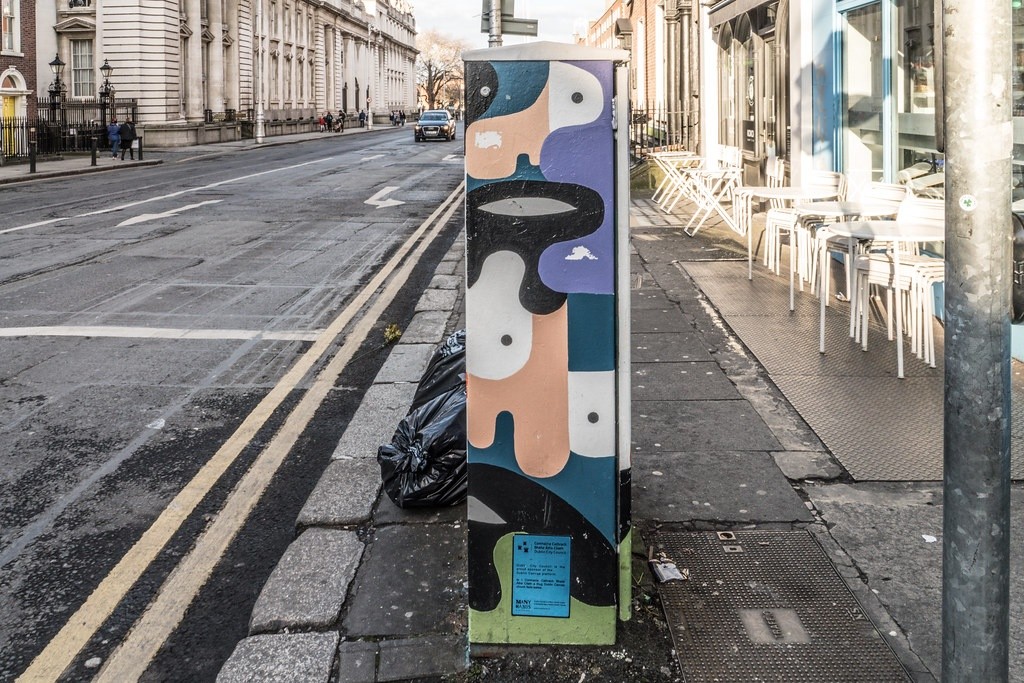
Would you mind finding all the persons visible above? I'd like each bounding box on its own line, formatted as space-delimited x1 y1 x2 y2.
390 110 406 126
117 118 136 160
359 110 366 127
338 110 346 132
320 113 325 132
325 112 333 132
107 118 121 159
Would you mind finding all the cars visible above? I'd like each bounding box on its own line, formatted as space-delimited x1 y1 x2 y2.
414 109 457 142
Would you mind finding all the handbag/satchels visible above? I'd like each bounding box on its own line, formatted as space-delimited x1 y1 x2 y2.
131 139 139 149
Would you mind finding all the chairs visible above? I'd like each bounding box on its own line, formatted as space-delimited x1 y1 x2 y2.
646 141 945 377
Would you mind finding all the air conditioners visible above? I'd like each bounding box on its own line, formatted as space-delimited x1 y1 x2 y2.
622 36 632 48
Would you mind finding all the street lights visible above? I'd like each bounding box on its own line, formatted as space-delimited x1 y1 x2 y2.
49 53 66 124
99 59 115 127
368 23 385 125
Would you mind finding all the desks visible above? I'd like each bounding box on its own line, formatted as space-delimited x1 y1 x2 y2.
789 201 895 312
748 188 836 282
648 150 696 203
819 220 944 378
660 156 712 213
679 169 746 237
